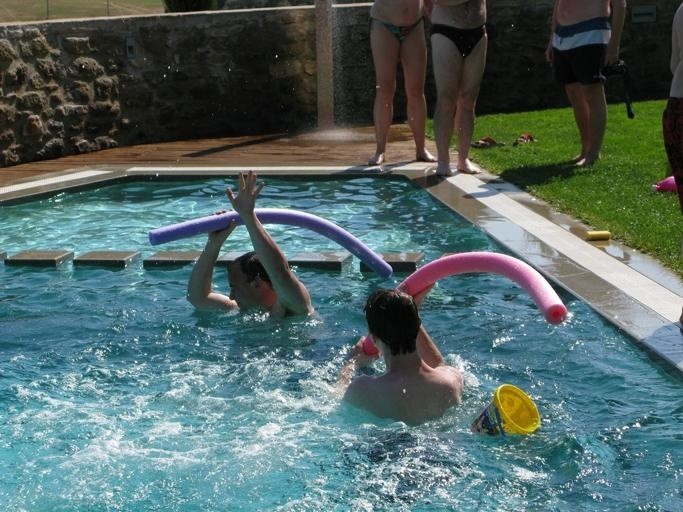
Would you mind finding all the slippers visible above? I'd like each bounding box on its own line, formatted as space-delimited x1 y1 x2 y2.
515 133 539 146
471 135 506 149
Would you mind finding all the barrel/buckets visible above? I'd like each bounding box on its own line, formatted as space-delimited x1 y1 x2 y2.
474 384 541 436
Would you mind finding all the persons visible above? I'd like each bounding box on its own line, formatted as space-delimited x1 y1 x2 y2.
429 1 489 177
367 0 438 167
544 0 627 171
661 4 683 214
185 170 312 323
335 289 463 428
